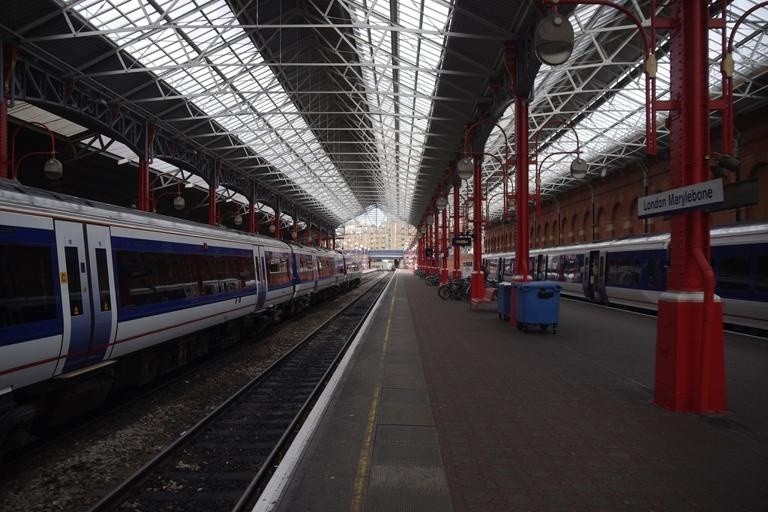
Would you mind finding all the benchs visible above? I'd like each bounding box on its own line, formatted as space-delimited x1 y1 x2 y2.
470 287 496 311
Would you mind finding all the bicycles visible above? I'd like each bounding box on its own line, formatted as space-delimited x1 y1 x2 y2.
437 276 470 300
414 270 440 286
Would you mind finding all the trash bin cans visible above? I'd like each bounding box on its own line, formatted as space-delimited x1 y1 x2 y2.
514 281 562 334
497 282 511 322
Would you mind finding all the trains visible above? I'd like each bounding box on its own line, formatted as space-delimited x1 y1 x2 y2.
0 178 361 397
470 222 767 336
